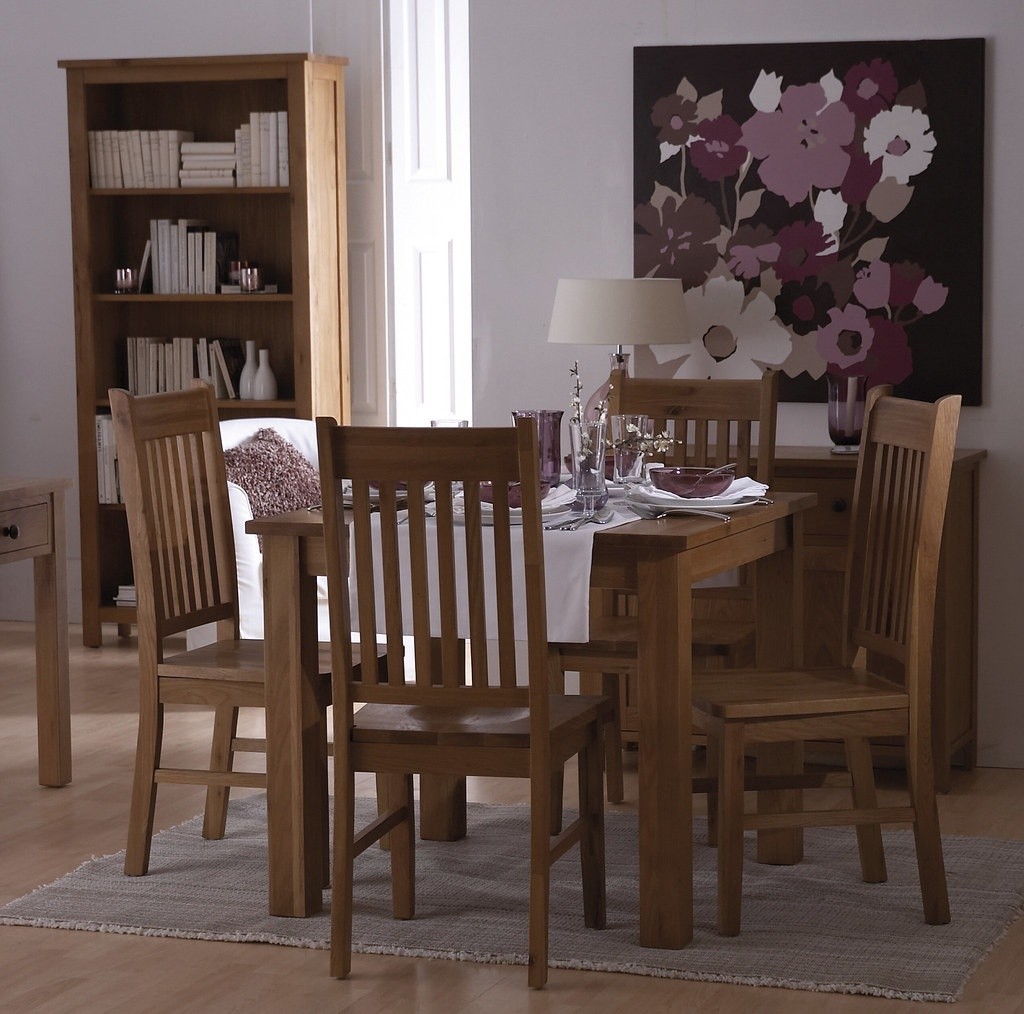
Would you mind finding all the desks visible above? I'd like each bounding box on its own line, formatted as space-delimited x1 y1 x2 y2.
0 479 74 788
244 475 820 950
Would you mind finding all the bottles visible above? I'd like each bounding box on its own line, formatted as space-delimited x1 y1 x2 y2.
238 340 258 400
254 349 278 400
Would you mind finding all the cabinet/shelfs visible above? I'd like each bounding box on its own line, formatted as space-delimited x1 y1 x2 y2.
564 446 988 793
57 54 352 649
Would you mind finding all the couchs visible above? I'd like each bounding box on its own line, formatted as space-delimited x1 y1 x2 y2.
188 418 416 682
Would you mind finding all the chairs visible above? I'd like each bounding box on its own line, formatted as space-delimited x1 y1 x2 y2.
315 417 608 991
690 383 963 937
106 383 406 875
542 368 778 846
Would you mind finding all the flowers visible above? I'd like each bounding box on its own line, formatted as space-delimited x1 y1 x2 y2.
570 360 682 475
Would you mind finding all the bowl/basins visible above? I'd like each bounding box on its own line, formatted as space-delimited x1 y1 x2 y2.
564 455 614 480
649 466 735 498
479 481 550 506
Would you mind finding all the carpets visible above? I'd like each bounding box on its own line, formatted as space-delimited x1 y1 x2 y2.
0 789 1024 1001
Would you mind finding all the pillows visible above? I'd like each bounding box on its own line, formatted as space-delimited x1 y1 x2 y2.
222 429 324 554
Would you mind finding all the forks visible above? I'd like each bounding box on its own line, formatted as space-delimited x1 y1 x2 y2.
627 504 731 523
569 497 594 531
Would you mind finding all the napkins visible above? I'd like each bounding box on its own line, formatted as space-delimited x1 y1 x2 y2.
627 476 768 500
455 483 577 512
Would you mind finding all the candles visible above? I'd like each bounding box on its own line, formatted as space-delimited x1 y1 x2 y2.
845 377 857 437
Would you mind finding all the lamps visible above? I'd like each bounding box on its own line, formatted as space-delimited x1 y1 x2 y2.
547 277 689 448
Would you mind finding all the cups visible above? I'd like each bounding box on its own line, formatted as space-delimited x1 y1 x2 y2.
512 409 565 486
611 414 648 482
114 267 137 294
570 422 608 498
225 260 249 285
824 374 869 446
239 268 261 293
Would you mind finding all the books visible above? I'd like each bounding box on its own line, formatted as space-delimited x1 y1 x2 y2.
126 337 239 399
88 111 289 189
113 585 137 606
136 218 237 294
95 415 126 504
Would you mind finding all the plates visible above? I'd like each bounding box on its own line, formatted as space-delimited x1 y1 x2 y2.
640 489 744 505
608 487 626 498
343 490 430 503
453 484 577 513
624 494 759 514
453 507 573 525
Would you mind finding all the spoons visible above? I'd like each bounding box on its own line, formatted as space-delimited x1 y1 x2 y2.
558 507 614 532
